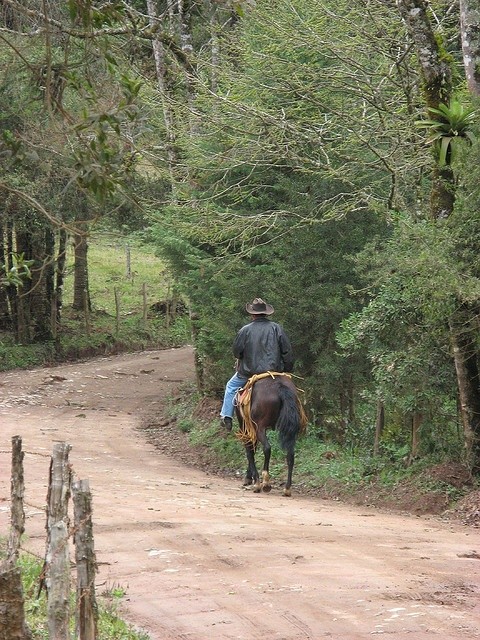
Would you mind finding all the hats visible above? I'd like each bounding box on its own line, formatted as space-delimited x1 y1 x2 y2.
246 298 274 315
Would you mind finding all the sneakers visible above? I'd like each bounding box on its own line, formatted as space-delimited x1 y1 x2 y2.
220 417 232 430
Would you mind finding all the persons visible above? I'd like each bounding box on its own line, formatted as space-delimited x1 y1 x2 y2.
219 297 295 431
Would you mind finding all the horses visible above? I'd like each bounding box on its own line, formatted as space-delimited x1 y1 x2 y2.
233 358 301 496
